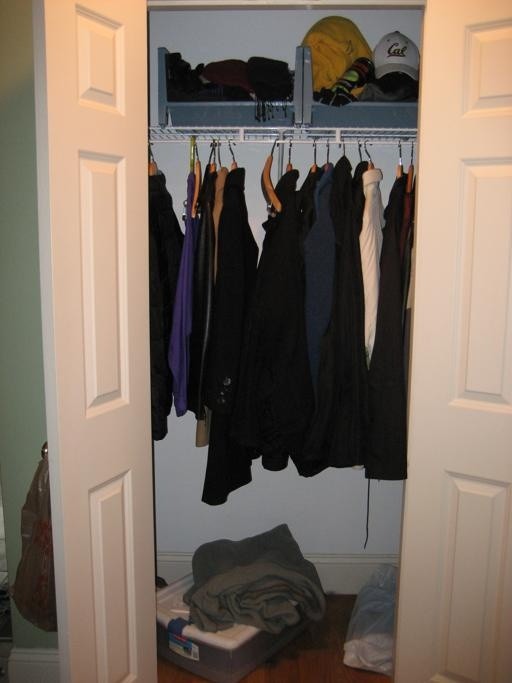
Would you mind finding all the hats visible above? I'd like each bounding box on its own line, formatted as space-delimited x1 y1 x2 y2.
374 30 426 83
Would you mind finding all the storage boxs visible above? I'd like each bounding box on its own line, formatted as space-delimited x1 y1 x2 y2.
155 572 307 683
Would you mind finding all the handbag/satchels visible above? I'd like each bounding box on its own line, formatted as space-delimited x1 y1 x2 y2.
13 459 59 633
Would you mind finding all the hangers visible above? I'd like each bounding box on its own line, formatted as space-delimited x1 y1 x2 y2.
150 126 416 220
